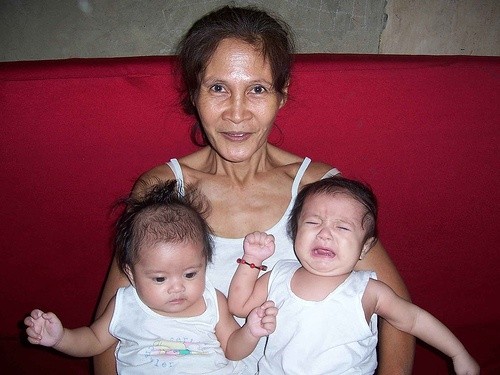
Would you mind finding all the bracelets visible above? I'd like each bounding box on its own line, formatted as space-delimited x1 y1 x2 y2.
235 257 269 273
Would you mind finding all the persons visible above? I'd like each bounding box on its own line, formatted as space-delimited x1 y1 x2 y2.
24 179 278 375
90 4 415 375
226 176 481 375
